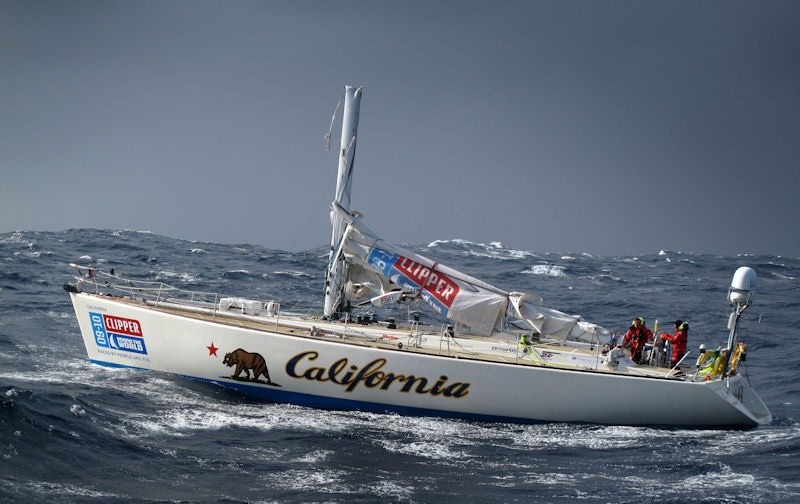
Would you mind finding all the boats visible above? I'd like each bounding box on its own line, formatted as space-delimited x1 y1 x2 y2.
63 85 773 430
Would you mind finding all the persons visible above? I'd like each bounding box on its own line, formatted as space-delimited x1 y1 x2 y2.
660 319 689 369
622 317 653 364
602 343 611 356
343 300 352 323
387 319 396 329
